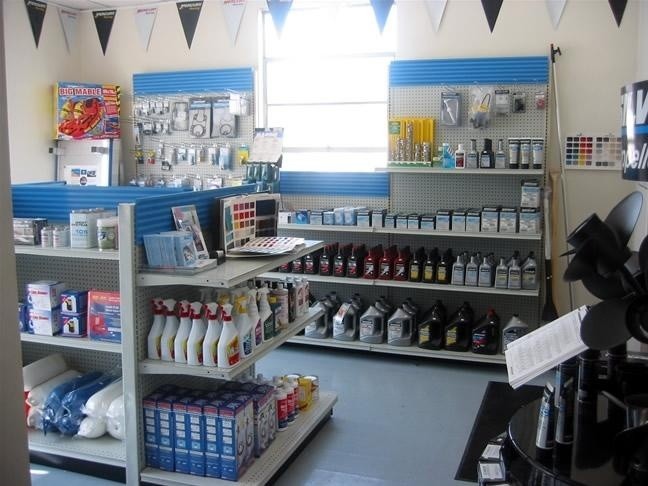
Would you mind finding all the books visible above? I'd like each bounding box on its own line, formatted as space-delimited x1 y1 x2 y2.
142 231 218 275
504 305 591 390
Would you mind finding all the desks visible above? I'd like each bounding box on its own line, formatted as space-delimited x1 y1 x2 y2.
452 378 647 485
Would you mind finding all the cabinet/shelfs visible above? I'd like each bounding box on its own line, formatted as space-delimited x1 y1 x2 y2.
128 183 337 486
9 184 137 484
258 161 548 372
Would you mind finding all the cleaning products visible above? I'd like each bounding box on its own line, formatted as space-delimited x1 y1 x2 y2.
144 272 310 370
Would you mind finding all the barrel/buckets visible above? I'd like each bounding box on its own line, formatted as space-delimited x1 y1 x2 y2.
303 286 529 355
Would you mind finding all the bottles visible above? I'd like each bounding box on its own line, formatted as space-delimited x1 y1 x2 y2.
40 224 69 249
246 276 311 332
274 241 538 292
433 138 506 170
251 372 299 432
394 123 430 163
555 342 628 446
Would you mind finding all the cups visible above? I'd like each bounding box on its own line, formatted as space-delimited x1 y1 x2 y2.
625 392 648 428
